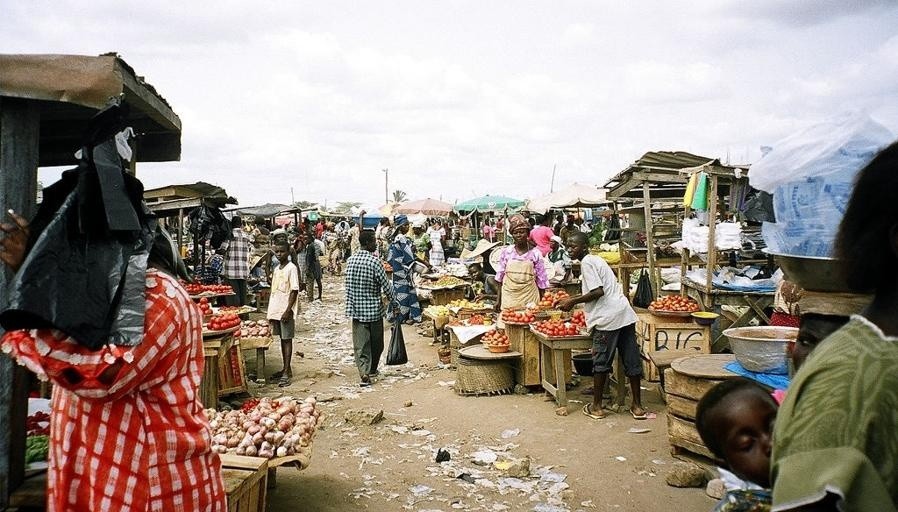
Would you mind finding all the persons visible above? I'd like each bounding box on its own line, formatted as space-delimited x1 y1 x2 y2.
772 313 848 407
0 164 228 511
769 137 898 511
695 378 779 512
559 231 646 421
149 210 360 302
265 240 300 389
346 230 402 386
374 210 623 322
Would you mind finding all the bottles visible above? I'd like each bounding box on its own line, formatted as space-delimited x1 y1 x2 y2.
481 298 494 326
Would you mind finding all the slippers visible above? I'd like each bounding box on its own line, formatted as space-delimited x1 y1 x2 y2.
581 402 606 421
629 405 648 420
359 369 379 387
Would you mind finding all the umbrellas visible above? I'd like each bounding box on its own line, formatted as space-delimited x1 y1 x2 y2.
454 194 525 246
398 198 455 217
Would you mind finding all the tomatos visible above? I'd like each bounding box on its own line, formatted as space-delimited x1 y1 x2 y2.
185 283 233 295
196 296 213 315
207 314 241 337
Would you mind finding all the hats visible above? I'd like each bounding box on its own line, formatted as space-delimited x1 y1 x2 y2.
393 214 409 228
508 214 531 236
464 237 503 260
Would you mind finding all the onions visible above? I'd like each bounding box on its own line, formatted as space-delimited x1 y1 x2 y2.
202 396 323 459
240 320 272 339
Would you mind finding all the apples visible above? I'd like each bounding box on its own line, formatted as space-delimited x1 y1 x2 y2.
502 290 586 336
481 329 510 346
651 294 700 312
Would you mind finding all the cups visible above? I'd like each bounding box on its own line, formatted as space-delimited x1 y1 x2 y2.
532 310 562 322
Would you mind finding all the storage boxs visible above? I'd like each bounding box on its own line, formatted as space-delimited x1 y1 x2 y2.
503 322 571 387
636 312 712 382
219 453 269 512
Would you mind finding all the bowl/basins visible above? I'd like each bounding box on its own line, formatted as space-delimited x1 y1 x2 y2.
571 352 594 376
759 245 846 293
487 344 510 353
691 311 720 325
648 307 698 319
722 325 800 375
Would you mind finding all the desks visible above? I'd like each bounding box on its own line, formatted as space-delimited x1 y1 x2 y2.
422 306 449 345
239 329 272 383
531 330 625 407
203 337 248 409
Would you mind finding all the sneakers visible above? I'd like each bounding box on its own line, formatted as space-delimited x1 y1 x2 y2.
271 369 293 387
